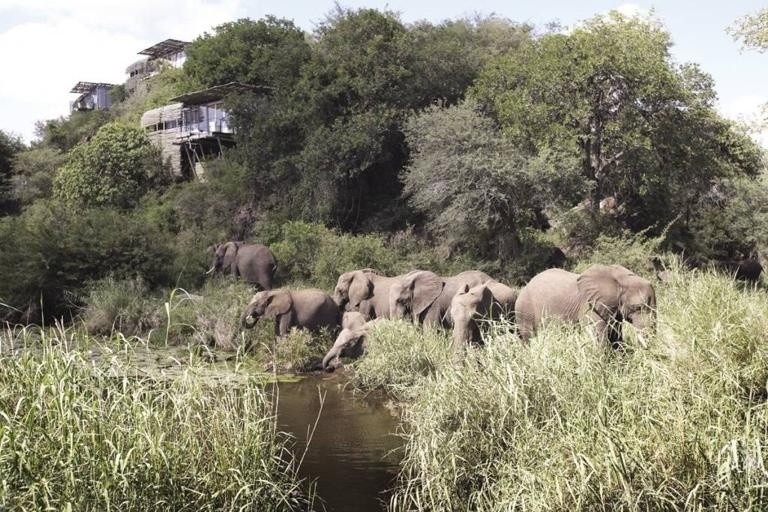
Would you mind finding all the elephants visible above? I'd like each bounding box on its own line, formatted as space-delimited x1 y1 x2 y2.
322 265 656 373
204 242 277 290
241 288 340 338
